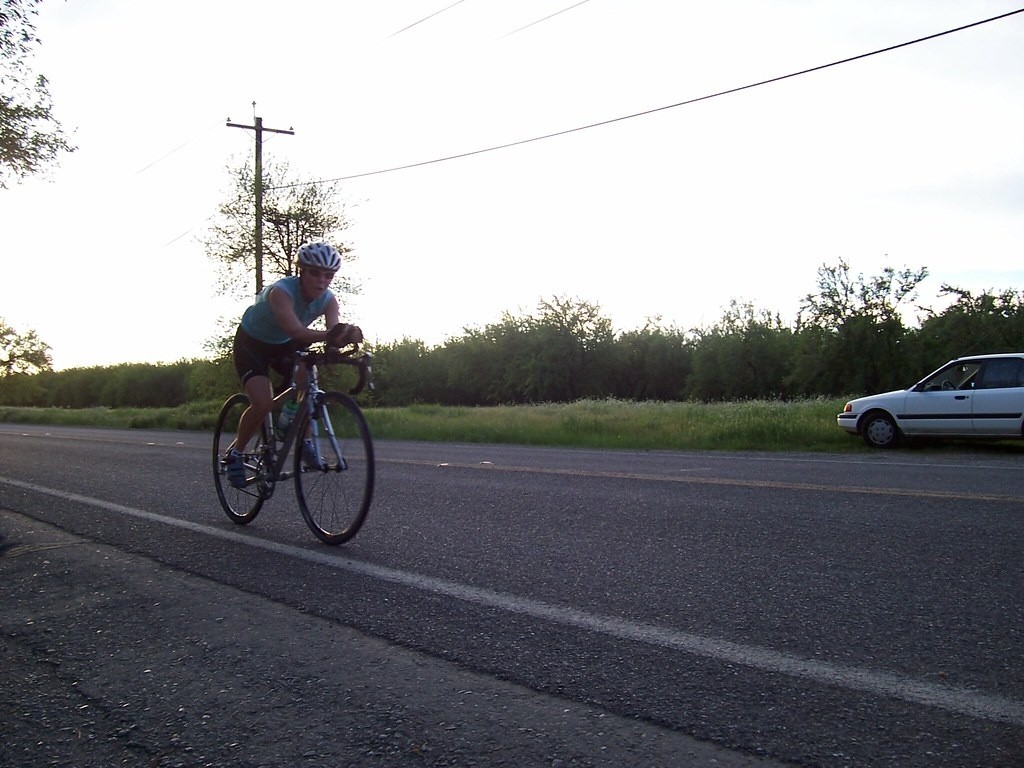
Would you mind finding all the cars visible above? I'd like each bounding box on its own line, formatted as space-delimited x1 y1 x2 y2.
838 353 1024 448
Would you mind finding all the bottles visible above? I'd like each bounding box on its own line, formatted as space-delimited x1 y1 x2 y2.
273 398 299 442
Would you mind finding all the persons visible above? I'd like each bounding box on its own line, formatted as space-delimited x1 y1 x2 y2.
225 244 363 487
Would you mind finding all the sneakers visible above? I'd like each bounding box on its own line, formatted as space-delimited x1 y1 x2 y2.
225 448 246 488
301 439 328 469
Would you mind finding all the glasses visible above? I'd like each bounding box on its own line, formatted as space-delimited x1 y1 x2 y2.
308 270 334 279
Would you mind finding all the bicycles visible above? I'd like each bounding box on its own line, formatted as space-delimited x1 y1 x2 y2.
211 344 377 545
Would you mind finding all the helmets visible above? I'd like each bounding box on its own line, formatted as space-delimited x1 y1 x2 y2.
292 242 341 272
